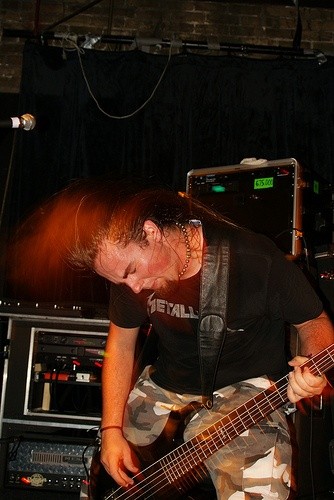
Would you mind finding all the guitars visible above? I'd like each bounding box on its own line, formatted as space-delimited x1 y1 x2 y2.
87 344 334 500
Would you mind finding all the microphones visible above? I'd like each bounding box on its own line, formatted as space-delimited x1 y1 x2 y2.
0 114 36 131
